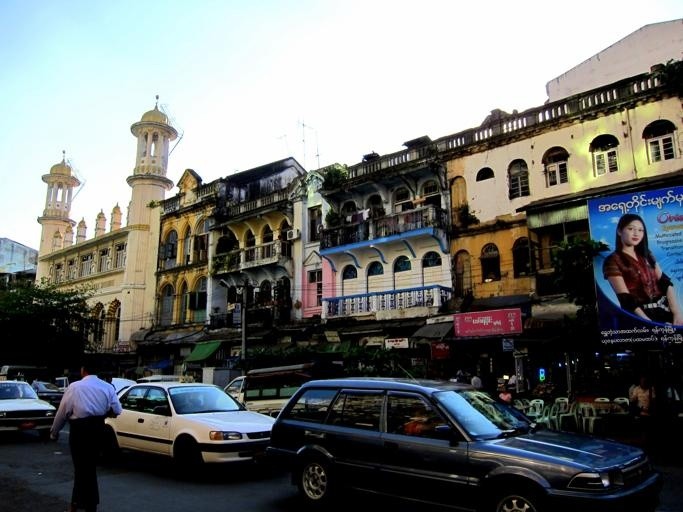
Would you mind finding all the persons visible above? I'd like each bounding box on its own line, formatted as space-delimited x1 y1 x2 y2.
449 363 683 465
401 411 445 437
47 359 123 512
601 212 683 326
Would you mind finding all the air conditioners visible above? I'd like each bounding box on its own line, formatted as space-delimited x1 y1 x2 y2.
287 228 301 240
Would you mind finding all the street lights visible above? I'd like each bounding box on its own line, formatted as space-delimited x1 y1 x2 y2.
219 279 248 376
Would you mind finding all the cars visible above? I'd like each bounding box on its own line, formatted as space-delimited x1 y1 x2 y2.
100 381 276 480
0 365 70 442
224 373 327 419
262 376 659 512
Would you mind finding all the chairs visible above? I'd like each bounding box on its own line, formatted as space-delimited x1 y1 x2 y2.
513 397 631 432
